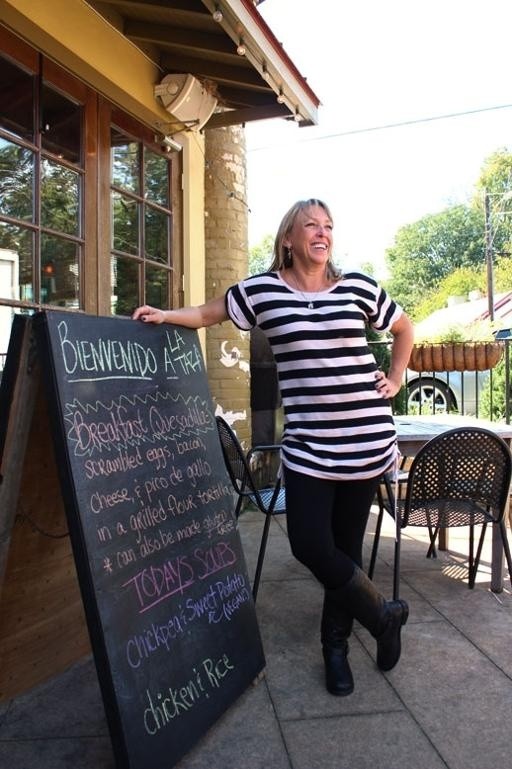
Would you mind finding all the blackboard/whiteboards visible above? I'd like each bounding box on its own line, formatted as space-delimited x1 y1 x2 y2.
46 309 267 767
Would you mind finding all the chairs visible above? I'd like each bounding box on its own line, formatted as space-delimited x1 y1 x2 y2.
217 415 286 606
369 427 512 601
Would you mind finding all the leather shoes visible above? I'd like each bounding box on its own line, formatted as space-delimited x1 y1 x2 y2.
321 632 355 696
377 600 408 671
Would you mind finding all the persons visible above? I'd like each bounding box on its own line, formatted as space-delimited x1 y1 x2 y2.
131 199 414 697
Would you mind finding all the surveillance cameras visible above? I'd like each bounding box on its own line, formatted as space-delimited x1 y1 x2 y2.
156 134 182 155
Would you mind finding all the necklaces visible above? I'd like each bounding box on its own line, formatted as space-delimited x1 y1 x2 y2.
287 270 325 309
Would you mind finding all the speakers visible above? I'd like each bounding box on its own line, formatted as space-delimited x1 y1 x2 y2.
161 73 217 133
